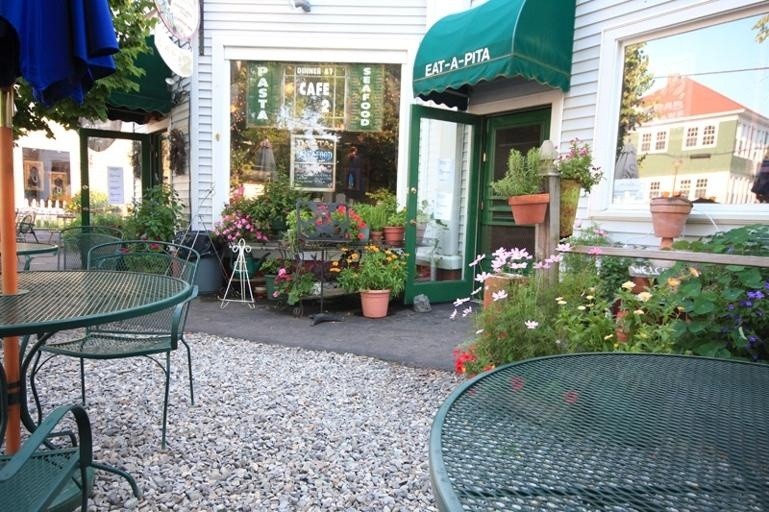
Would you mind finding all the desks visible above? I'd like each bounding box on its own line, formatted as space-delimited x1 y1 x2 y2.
0 241 58 271
0 270 192 499
429 352 769 512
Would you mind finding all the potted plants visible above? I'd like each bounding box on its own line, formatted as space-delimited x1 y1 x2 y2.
489 145 550 224
258 257 290 301
384 207 408 245
360 204 387 245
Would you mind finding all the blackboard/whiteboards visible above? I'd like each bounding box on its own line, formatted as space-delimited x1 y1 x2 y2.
289 134 337 193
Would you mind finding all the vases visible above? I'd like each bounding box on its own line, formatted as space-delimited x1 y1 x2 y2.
560 175 582 239
651 197 692 238
358 287 392 319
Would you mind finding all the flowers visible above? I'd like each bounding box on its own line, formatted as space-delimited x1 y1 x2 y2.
554 136 608 196
328 245 409 300
213 209 270 242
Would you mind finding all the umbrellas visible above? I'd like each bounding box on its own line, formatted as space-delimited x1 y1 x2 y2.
0 0 121 454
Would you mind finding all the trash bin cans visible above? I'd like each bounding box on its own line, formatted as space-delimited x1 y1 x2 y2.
176 230 223 295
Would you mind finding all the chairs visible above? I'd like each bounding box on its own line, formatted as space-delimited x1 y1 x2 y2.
0 404 96 511
38 240 201 450
16 207 40 243
54 226 125 334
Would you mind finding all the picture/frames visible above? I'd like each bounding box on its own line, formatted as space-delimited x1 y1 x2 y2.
24 161 44 192
49 172 67 201
